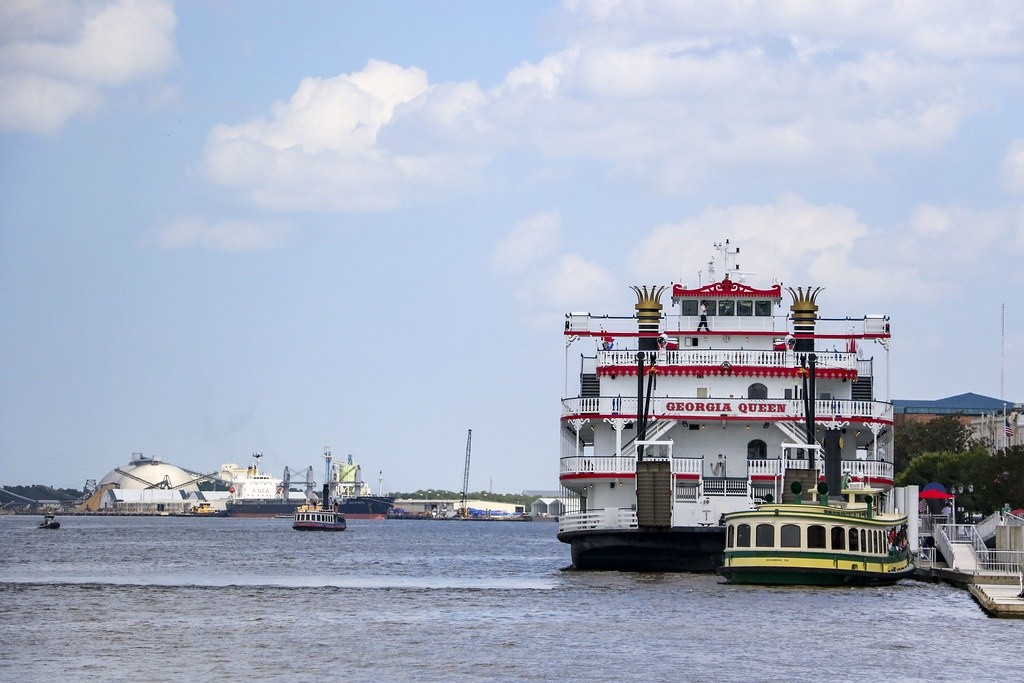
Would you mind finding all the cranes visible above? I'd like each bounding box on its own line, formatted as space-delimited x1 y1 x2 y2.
453 429 472 519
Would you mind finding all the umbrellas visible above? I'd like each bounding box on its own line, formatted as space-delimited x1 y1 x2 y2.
919 489 954 531
1010 509 1024 518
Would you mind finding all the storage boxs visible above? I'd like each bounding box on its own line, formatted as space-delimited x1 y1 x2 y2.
849 482 864 490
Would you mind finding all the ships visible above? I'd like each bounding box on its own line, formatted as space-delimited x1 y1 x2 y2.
554 235 894 570
225 448 396 522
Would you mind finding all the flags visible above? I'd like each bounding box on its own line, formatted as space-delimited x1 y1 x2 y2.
1005 418 1014 437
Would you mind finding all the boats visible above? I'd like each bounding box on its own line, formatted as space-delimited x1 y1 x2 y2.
36 512 62 530
291 483 349 531
721 471 916 587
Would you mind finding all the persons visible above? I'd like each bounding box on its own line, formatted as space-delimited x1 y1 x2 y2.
919 499 952 528
878 459 886 475
887 525 910 556
696 300 712 332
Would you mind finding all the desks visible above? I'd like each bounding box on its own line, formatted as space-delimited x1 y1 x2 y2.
808 488 884 508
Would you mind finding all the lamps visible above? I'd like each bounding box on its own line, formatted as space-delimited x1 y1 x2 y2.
764 422 769 428
682 421 688 427
658 333 669 349
784 335 796 351
